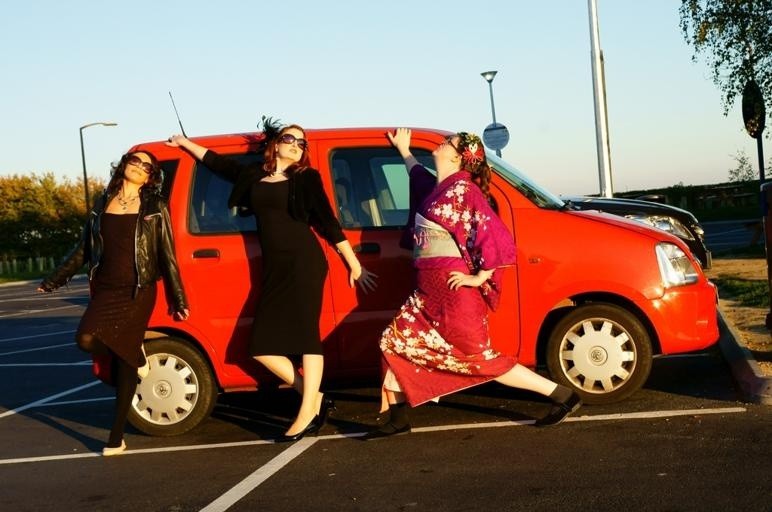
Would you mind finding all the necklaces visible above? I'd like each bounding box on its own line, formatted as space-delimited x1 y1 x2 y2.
113 191 140 212
271 169 285 175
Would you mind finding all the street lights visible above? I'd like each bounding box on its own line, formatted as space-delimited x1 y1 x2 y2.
79 119 118 268
479 65 503 157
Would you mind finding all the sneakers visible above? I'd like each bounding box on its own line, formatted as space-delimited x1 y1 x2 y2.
364 421 411 442
100 439 126 456
535 392 585 427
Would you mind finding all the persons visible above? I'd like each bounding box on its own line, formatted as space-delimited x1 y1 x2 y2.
36 150 190 456
163 117 382 444
360 127 584 443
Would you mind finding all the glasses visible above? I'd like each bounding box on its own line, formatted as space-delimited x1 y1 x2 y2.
128 155 154 174
277 133 308 152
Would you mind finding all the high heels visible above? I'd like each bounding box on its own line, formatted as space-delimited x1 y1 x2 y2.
315 394 338 432
279 413 321 444
